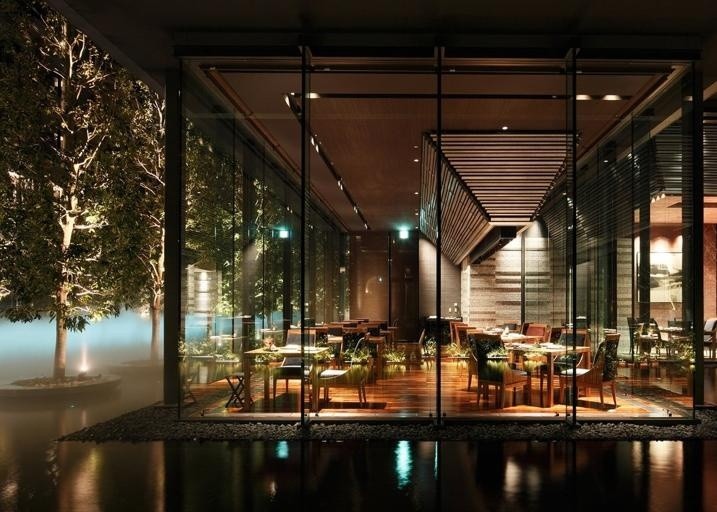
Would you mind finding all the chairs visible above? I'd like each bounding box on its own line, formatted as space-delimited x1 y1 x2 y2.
176 314 716 411
